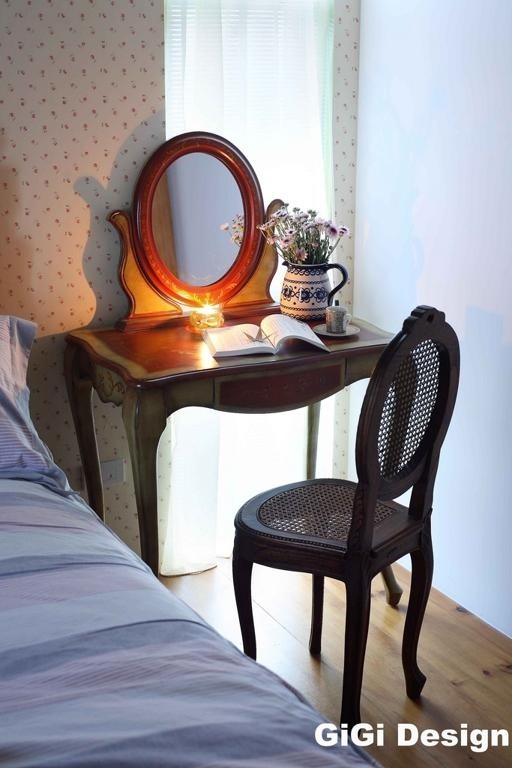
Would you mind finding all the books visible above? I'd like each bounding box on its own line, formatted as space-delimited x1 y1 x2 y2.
202 314 331 358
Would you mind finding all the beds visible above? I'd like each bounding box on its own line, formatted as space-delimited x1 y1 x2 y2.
0 312 388 768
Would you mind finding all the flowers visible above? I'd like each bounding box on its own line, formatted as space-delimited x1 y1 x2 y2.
255 203 349 263
217 213 245 248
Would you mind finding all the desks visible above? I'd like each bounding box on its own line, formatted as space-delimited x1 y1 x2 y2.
65 305 419 607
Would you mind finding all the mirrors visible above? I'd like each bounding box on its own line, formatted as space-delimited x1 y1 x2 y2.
129 129 266 311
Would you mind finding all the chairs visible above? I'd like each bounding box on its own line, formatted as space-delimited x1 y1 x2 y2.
232 304 461 729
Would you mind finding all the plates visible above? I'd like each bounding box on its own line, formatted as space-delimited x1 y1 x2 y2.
312 324 359 336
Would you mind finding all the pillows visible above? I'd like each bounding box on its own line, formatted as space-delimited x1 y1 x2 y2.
0 312 80 497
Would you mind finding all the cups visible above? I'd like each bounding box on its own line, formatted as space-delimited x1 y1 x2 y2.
326 307 353 333
